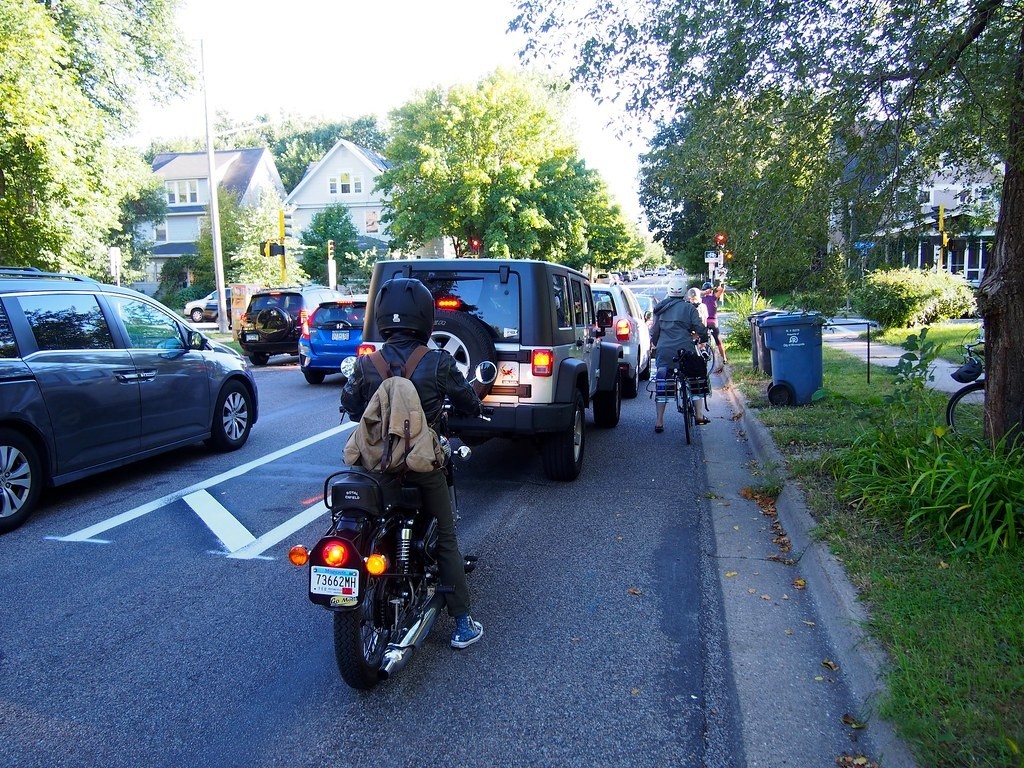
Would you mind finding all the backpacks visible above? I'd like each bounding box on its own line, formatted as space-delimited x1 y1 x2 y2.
341 346 445 477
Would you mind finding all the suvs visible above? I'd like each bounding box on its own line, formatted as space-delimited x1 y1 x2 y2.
358 259 625 481
0 264 260 540
589 281 655 399
238 285 349 365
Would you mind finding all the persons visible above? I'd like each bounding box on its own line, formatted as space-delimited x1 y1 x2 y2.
700 278 729 365
649 278 712 432
340 278 486 648
687 285 710 360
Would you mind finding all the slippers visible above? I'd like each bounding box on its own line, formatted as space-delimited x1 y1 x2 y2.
695 415 711 425
656 426 664 431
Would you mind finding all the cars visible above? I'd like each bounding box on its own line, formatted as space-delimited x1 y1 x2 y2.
658 267 667 276
296 294 371 383
613 269 670 332
674 269 684 276
643 268 654 276
596 272 614 286
182 286 233 326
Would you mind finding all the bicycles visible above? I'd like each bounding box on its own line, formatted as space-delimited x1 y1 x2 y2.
943 322 987 447
675 325 716 445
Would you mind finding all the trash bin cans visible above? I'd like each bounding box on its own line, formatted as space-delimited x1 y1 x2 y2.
747 307 827 406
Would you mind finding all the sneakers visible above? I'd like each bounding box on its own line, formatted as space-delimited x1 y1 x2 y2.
452 617 484 648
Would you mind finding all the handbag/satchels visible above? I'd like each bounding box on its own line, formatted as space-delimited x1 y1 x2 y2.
679 348 710 396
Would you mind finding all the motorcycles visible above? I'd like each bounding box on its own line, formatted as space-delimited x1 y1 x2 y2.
286 356 498 687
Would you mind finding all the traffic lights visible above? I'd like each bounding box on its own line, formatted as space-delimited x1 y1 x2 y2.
258 241 271 258
326 240 337 259
468 226 481 251
949 238 967 252
715 233 727 246
930 204 944 232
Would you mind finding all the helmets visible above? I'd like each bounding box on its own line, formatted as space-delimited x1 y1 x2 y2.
668 279 686 297
687 287 701 299
702 282 713 290
375 278 435 343
950 358 982 383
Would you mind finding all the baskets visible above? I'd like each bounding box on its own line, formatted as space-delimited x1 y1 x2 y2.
686 377 712 397
654 376 677 404
